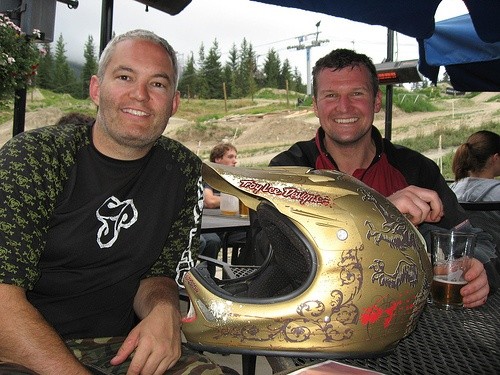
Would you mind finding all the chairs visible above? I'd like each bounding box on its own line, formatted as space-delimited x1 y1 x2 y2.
459 201 500 272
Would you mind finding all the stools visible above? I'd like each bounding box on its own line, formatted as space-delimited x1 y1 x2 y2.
220 241 243 263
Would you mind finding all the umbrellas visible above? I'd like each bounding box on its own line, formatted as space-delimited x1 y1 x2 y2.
417 12 500 93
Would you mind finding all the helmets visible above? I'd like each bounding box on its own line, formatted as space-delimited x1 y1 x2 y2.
181 160 433 359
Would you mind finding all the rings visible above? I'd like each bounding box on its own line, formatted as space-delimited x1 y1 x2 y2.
484 299 486 303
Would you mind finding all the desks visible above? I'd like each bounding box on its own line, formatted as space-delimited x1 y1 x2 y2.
224 264 500 375
200 208 250 235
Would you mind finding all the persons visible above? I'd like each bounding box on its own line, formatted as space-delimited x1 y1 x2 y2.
203 143 238 208
254 48 500 308
448 130 500 263
0 30 202 375
57 112 94 126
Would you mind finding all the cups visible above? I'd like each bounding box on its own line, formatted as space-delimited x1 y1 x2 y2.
432 230 477 311
219 193 240 216
